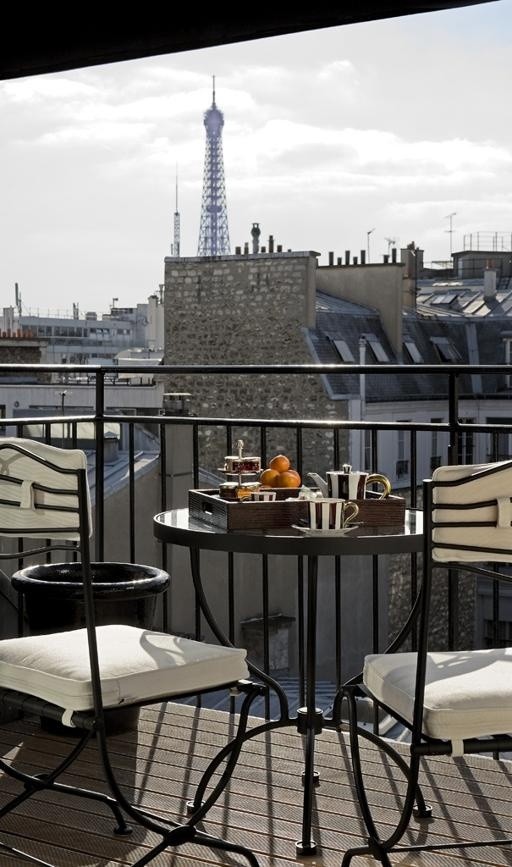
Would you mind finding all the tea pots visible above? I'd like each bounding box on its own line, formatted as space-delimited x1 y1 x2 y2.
307 462 392 501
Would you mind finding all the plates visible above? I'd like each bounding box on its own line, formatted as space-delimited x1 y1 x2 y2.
290 521 358 537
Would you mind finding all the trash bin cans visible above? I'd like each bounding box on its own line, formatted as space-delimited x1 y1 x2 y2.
11 561 171 738
241 612 297 673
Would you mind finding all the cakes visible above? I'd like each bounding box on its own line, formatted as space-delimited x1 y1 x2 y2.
219 481 262 499
225 456 261 473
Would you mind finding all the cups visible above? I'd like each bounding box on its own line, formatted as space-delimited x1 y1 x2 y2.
309 497 360 529
